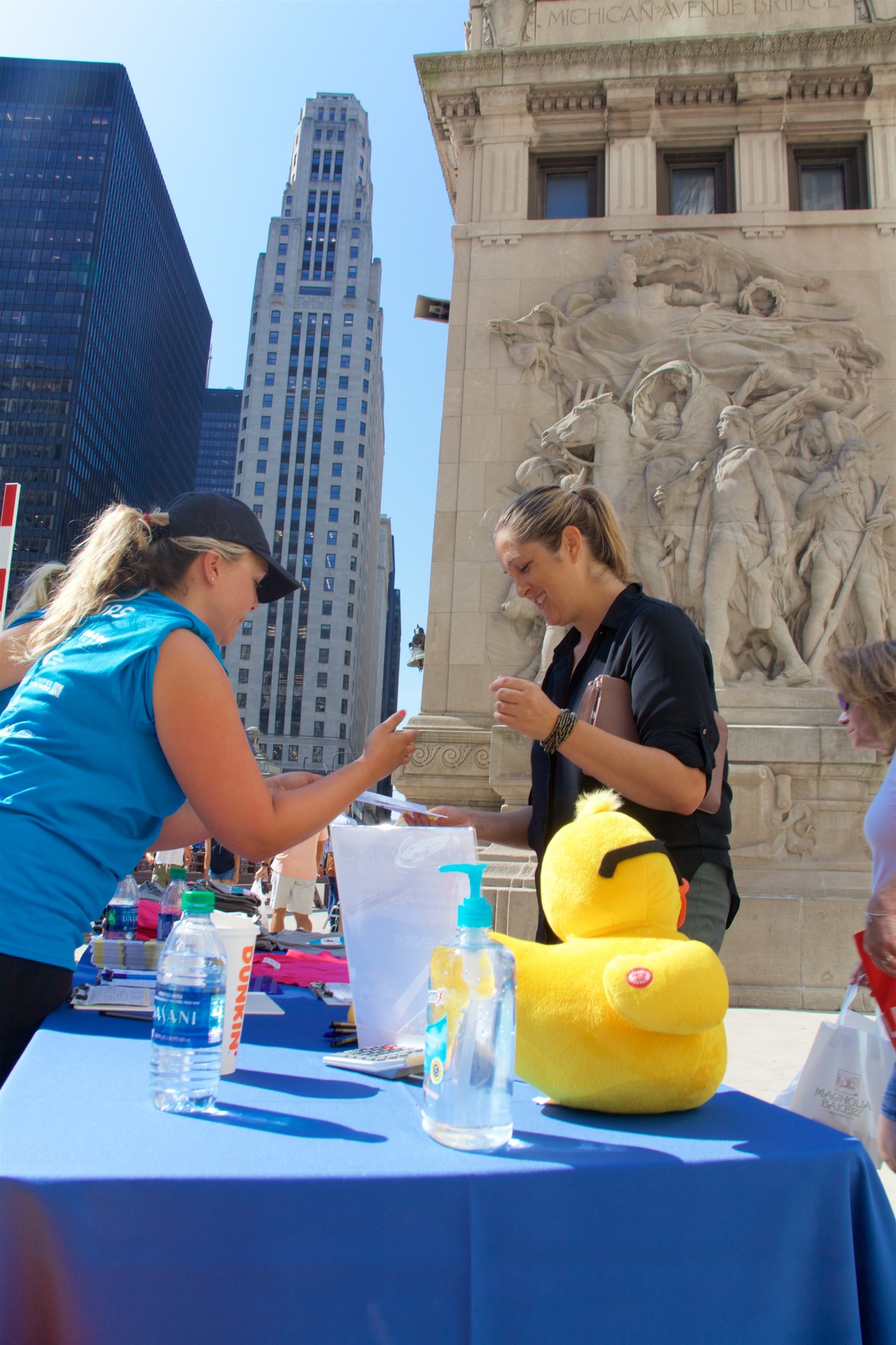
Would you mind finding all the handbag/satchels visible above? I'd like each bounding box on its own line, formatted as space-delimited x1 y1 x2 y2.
769 932 896 1173
577 676 728 815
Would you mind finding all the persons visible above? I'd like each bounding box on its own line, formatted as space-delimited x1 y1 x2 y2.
145 846 193 891
253 826 330 934
399 485 741 959
0 563 69 727
204 837 241 886
317 801 362 933
818 640 896 1173
0 489 419 1083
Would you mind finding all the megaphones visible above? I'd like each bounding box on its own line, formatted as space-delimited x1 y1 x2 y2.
413 295 450 324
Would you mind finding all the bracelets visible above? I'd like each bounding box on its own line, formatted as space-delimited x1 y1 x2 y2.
539 707 579 756
261 863 269 867
863 912 894 924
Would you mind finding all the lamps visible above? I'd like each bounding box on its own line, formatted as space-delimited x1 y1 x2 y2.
407 624 426 672
413 294 450 326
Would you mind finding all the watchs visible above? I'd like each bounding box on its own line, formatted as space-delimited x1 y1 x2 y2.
183 866 188 869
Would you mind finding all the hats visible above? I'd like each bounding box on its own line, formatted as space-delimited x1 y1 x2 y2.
146 490 303 605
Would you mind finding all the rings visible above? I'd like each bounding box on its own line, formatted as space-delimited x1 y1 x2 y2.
882 955 894 964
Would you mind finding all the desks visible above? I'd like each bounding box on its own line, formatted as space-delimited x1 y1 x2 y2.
0 945 895 1344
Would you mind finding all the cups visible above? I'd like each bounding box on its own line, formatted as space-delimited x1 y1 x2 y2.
173 908 261 1076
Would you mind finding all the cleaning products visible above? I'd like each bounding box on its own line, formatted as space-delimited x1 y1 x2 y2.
417 858 520 1153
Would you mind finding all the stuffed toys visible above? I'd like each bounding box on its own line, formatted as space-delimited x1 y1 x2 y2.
457 788 730 1117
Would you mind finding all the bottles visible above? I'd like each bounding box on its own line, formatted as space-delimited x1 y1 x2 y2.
149 891 228 1114
155 866 190 969
104 868 140 941
